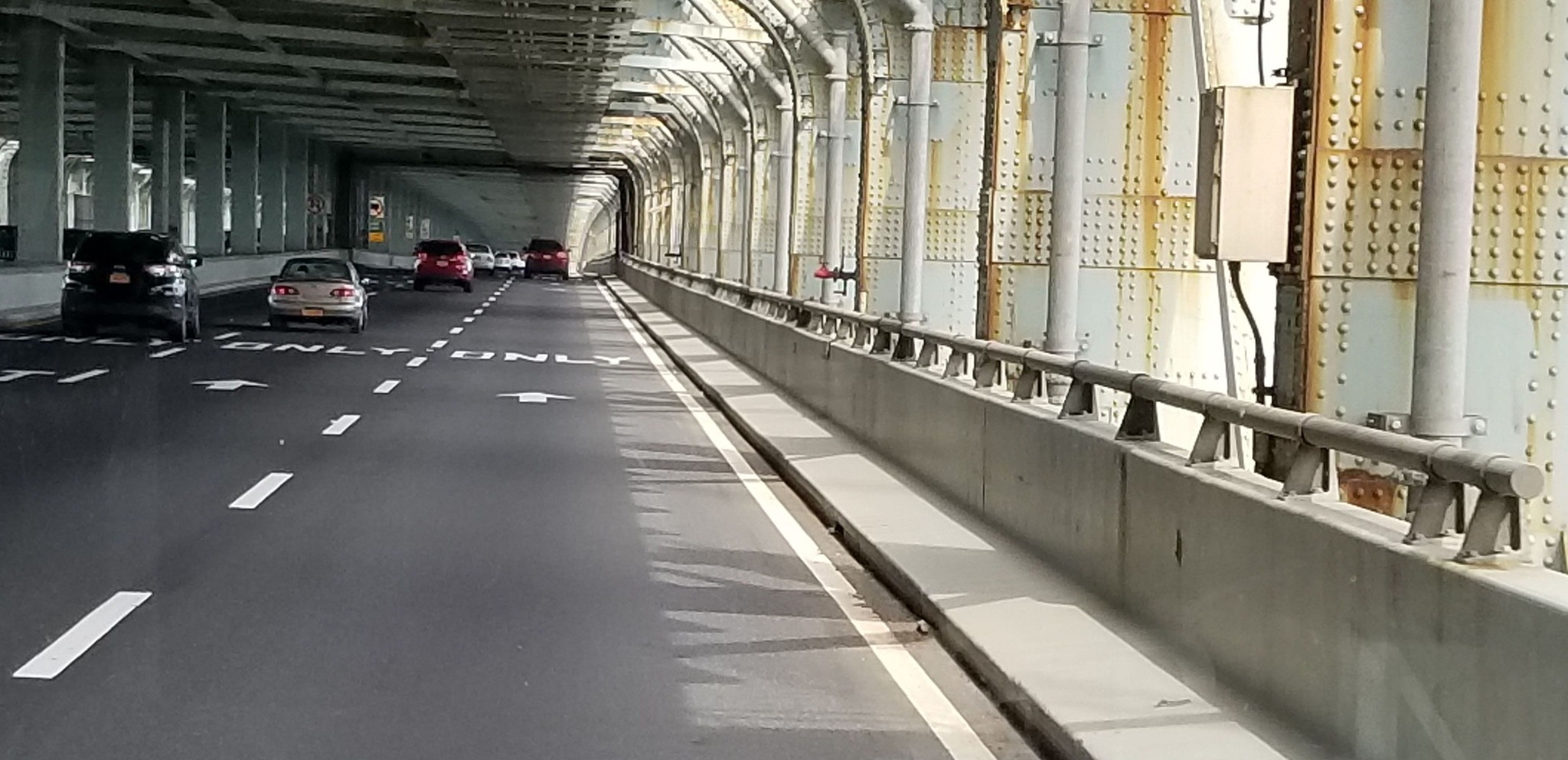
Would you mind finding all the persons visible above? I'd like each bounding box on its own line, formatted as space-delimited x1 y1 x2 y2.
298 265 310 274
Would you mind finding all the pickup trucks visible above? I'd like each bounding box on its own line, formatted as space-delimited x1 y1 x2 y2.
523 237 572 280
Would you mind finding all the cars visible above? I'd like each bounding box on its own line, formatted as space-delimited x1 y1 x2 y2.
265 257 371 334
494 248 526 274
412 238 482 293
466 242 495 277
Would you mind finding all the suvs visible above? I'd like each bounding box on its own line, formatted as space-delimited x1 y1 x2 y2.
59 228 204 346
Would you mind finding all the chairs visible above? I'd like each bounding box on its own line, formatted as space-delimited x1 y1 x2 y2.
297 264 309 275
